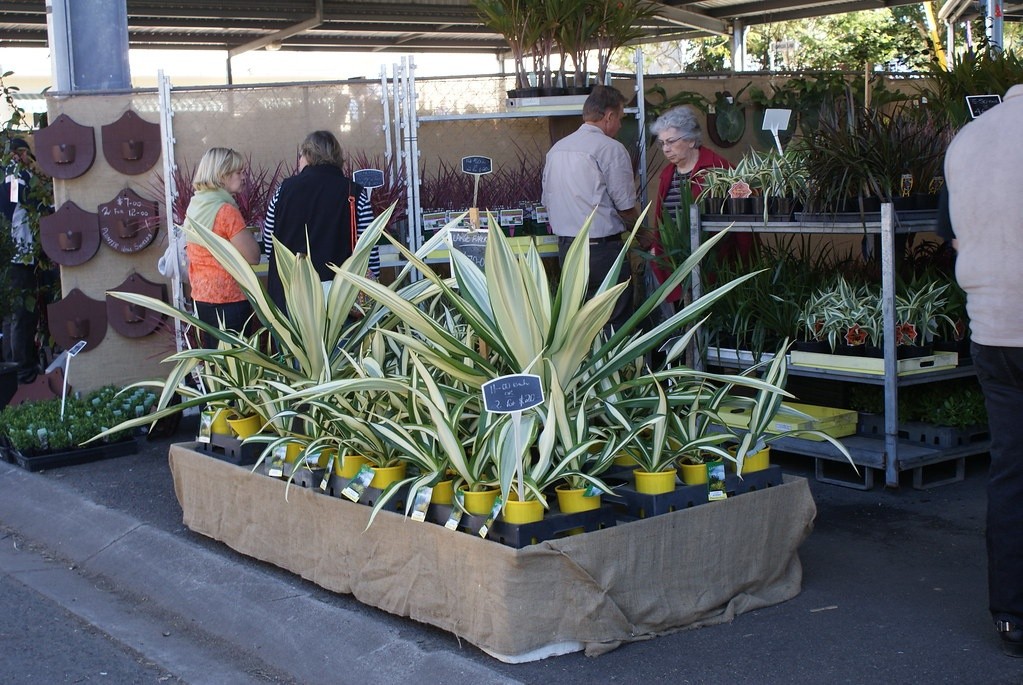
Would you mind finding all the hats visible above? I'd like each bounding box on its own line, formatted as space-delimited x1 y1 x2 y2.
9 138 36 161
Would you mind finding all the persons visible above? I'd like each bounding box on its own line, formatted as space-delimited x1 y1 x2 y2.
0 139 61 385
541 85 654 344
943 82 1023 660
648 105 763 369
264 130 382 371
179 149 270 358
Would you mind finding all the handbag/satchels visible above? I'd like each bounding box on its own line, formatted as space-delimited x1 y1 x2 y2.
158 225 188 284
348 268 380 318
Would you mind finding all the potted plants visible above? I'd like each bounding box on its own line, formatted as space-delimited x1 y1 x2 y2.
0 0 993 549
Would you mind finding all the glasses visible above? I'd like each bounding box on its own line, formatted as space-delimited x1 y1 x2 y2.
656 134 689 150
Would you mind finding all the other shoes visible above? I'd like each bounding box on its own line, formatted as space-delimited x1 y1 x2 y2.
997 621 1023 657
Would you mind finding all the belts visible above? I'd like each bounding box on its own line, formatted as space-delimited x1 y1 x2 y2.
559 233 622 244
